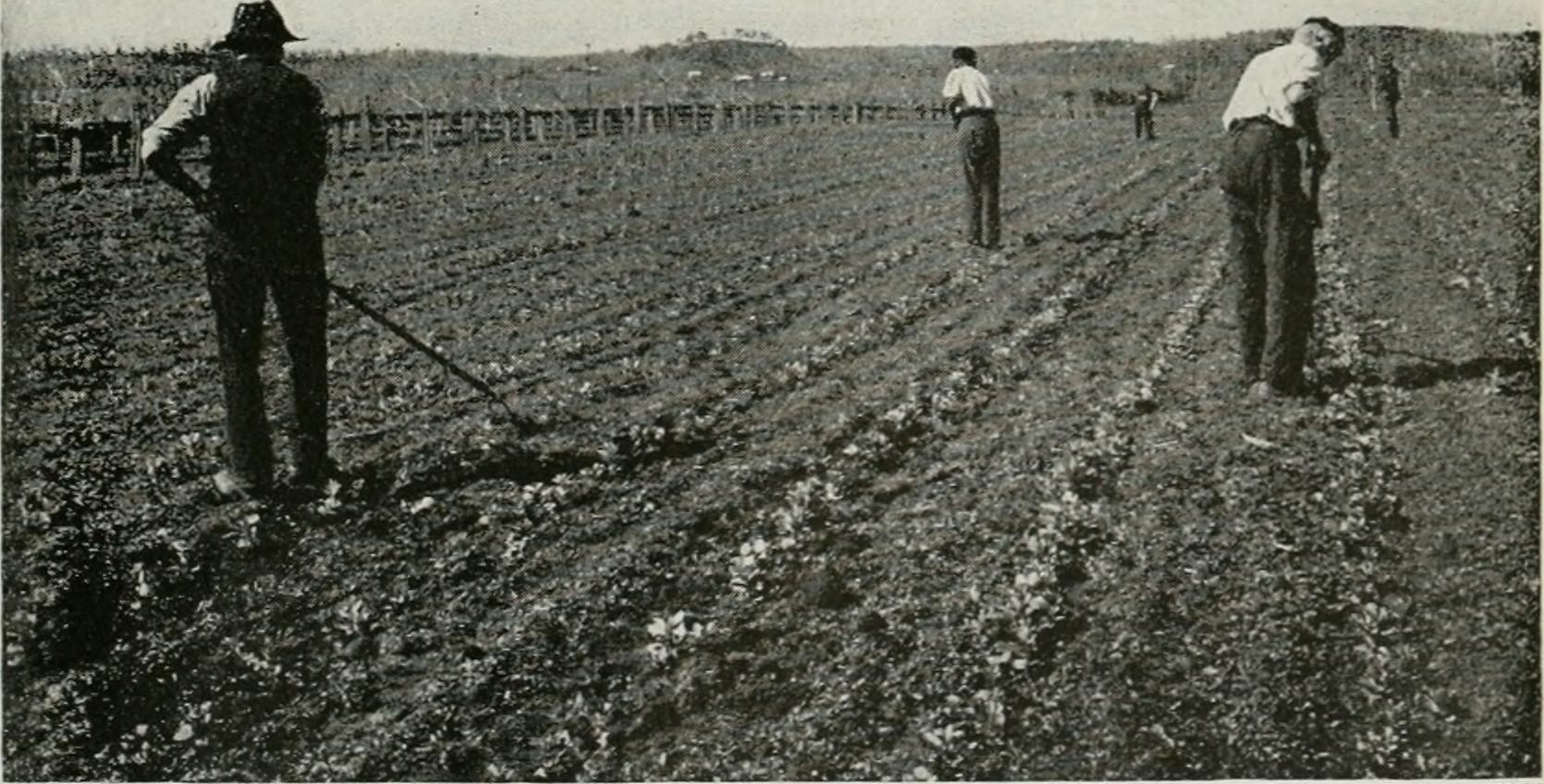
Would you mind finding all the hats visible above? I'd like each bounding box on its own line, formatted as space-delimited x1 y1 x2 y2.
211 1 308 52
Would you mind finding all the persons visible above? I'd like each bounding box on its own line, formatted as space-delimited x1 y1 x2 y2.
1135 88 1161 139
1378 51 1400 137
943 45 1004 248
138 0 331 503
1221 16 1347 395
